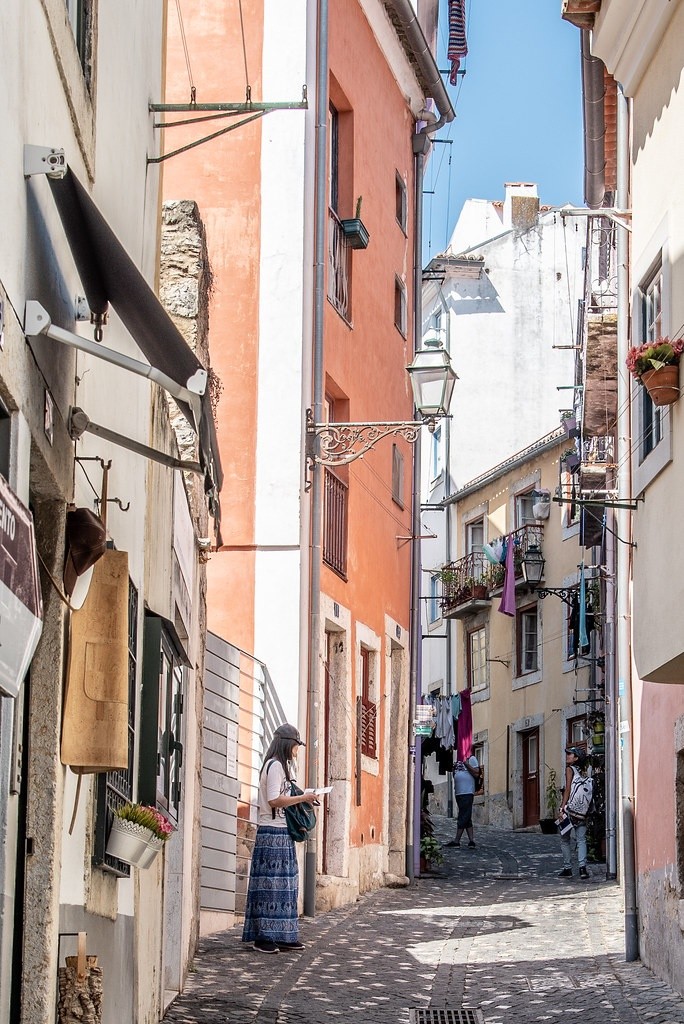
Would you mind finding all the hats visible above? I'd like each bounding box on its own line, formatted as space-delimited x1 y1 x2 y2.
273 723 306 747
565 747 587 760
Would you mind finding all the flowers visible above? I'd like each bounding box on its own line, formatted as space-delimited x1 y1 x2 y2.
140 805 175 841
625 335 684 387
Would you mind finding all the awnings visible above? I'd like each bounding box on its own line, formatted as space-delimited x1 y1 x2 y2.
25 145 226 502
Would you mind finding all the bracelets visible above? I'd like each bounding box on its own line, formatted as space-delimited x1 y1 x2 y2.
558 807 563 813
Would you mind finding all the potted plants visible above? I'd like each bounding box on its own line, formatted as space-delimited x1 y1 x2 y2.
420 837 445 873
538 762 562 835
588 819 606 859
105 800 161 865
438 569 490 609
561 411 577 440
560 447 580 473
581 710 606 735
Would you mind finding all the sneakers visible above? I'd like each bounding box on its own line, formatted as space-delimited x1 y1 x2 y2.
468 842 475 848
579 865 589 878
557 868 572 878
443 841 460 848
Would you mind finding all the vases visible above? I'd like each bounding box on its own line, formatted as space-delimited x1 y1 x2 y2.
119 834 164 870
641 366 679 406
592 734 601 744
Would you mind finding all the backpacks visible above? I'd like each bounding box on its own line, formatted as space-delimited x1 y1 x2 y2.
467 755 483 793
563 766 594 819
267 759 316 842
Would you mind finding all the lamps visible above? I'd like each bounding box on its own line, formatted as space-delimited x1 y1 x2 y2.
340 195 371 251
304 337 460 494
520 543 602 628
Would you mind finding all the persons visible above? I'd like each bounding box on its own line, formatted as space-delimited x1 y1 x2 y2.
557 747 594 879
443 754 480 848
242 725 320 953
